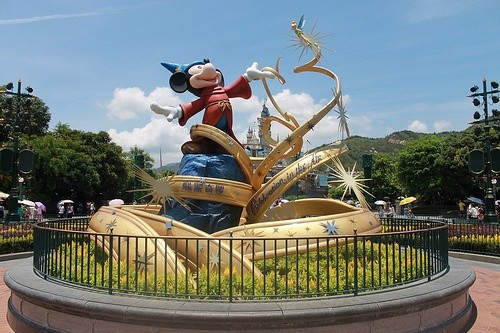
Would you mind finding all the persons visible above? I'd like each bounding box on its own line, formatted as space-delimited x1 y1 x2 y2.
17 204 42 222
347 199 362 208
378 204 396 217
133 199 137 205
459 200 484 220
496 202 500 218
58 203 74 219
274 198 289 206
86 201 95 216
404 203 414 218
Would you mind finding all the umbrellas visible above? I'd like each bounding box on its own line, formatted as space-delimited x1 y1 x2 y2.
467 197 485 205
61 200 74 203
17 200 35 206
374 200 386 205
35 202 46 211
400 197 416 205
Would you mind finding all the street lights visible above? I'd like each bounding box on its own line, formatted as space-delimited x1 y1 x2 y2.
1 77 38 226
467 75 500 224
